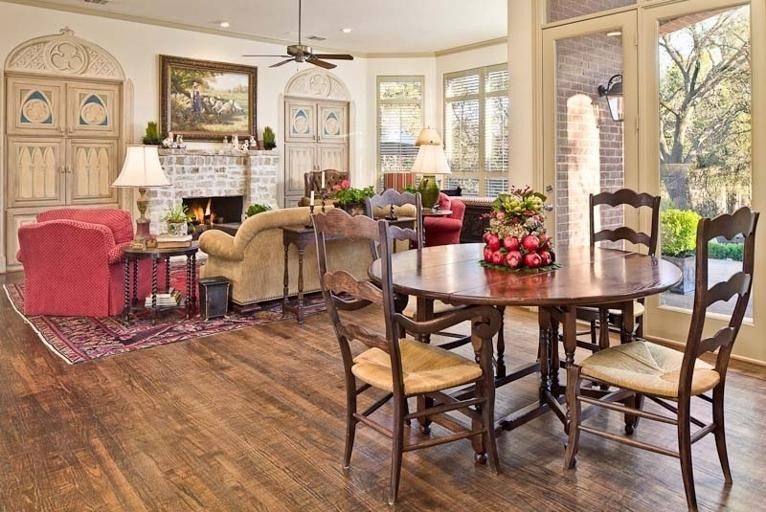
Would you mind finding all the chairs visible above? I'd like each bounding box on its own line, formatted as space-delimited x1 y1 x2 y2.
562 207 761 512
365 188 506 377
310 209 501 506
537 189 661 430
423 192 466 246
16 207 166 318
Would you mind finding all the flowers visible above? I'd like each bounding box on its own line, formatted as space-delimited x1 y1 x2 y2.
332 180 376 205
479 183 562 272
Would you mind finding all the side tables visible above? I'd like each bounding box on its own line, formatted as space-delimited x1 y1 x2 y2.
120 241 199 326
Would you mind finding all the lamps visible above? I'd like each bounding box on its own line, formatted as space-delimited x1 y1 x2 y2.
241 0 353 69
110 146 173 250
415 128 444 146
599 75 624 122
410 144 452 211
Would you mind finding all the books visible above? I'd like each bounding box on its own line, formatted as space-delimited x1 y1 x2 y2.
144 288 182 307
156 233 192 249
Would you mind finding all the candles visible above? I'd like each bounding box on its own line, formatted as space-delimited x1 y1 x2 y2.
310 190 315 206
321 171 326 188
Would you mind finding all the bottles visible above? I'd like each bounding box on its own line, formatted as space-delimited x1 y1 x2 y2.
223 136 227 148
232 135 237 149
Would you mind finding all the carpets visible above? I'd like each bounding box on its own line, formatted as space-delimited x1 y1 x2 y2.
3 260 358 366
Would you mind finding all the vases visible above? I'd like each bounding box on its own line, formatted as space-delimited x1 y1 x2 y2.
339 203 367 216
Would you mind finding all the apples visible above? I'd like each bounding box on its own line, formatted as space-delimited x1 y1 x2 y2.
483 231 555 269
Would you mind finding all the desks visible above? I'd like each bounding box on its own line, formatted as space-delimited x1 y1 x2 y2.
278 217 416 324
368 242 683 464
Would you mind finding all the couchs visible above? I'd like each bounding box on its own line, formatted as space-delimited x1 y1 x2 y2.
198 203 417 316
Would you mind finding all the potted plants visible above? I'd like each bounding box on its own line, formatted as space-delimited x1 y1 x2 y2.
263 126 276 149
660 208 704 294
160 204 189 238
143 121 162 146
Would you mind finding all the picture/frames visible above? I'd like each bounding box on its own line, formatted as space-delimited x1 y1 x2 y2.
158 54 257 140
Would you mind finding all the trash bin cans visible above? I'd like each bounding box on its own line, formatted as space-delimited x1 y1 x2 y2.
197 276 231 320
